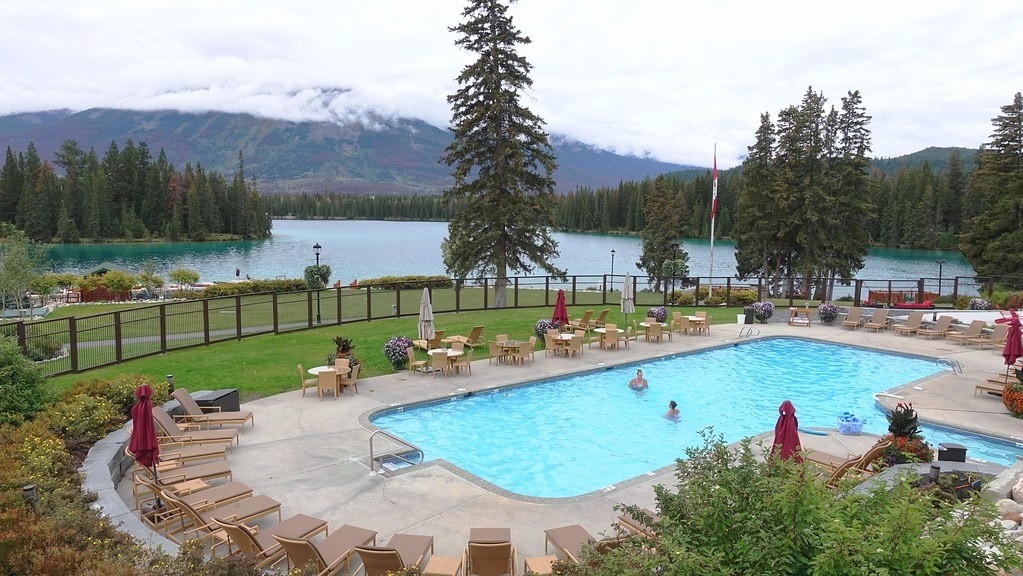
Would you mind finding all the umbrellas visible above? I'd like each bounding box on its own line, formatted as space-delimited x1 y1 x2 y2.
551 289 570 332
769 400 804 479
1002 319 1023 384
620 272 636 327
417 287 437 367
128 385 161 486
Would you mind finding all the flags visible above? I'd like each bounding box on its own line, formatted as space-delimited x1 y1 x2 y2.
710 155 719 218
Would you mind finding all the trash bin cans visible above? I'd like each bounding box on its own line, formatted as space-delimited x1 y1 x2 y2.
744 306 754 324
939 442 967 462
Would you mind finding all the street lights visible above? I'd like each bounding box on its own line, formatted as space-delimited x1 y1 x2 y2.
312 241 322 323
671 242 678 307
935 258 946 297
610 249 616 292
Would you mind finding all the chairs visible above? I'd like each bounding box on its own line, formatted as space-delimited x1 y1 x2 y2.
296 307 710 402
803 440 893 488
841 306 1023 397
124 388 673 576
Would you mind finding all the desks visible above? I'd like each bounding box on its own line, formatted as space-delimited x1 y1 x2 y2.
427 349 464 376
682 315 706 335
551 334 578 357
594 328 625 350
308 366 351 397
788 308 814 327
496 340 524 365
640 322 669 342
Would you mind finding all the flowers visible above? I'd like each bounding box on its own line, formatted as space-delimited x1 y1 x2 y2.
751 301 775 324
816 303 838 322
869 401 934 471
327 336 362 388
384 335 415 370
1000 381 1023 419
532 318 560 349
647 306 668 323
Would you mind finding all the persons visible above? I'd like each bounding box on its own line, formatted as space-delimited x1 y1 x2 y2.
667 400 679 416
628 370 648 389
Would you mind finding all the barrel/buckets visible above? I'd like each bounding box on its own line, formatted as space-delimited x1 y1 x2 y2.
744 306 754 324
737 314 746 324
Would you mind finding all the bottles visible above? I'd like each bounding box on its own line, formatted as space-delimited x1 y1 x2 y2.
933 312 936 321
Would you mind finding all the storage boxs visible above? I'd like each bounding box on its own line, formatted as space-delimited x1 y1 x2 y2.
836 416 863 436
